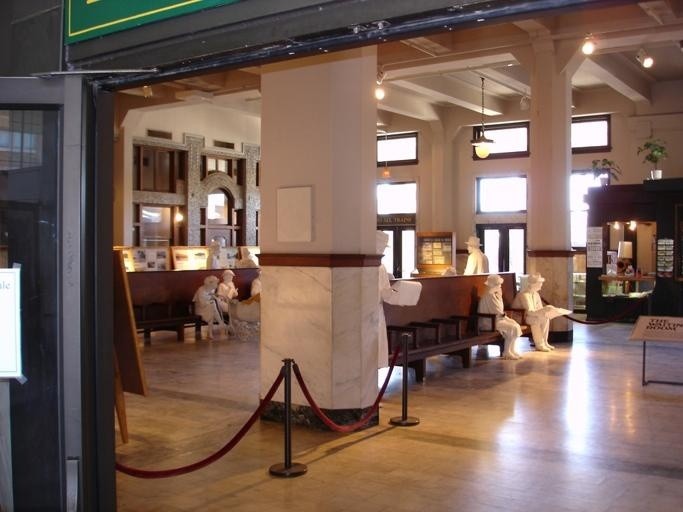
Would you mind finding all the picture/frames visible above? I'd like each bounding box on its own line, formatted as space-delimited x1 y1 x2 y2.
112 245 261 272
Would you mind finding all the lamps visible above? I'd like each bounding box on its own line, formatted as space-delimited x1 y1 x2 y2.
374 38 653 159
618 241 633 274
382 131 390 180
608 220 639 232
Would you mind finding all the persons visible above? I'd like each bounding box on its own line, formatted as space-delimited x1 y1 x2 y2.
191 275 226 340
375 229 395 371
133 249 141 262
139 251 146 261
233 247 258 268
463 236 489 275
205 241 222 269
476 275 523 361
513 271 556 352
218 270 239 313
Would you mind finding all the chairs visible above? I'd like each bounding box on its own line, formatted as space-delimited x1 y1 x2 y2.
133 296 249 345
386 307 546 383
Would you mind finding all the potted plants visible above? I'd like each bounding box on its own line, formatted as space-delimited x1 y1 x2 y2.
592 158 622 192
637 138 666 181
